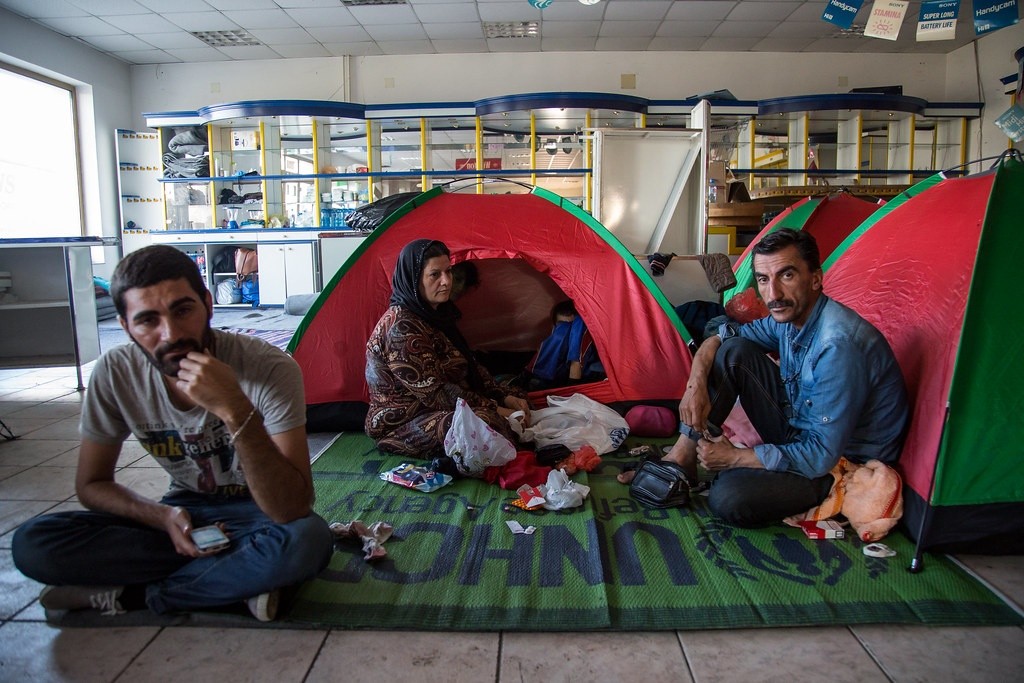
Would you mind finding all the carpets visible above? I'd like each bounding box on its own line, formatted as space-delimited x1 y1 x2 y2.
42 430 1024 632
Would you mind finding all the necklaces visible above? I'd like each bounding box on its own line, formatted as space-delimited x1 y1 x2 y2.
781 323 815 389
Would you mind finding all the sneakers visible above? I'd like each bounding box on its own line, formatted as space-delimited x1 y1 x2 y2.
41 583 127 615
245 588 283 622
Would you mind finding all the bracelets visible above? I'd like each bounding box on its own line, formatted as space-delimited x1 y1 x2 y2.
228 408 259 446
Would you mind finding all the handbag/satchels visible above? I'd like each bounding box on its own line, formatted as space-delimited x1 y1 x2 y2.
444 398 518 475
509 393 629 454
629 460 691 509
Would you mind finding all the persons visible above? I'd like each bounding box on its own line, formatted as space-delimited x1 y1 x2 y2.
538 295 581 390
11 244 333 623
364 239 531 451
616 228 912 529
435 260 481 352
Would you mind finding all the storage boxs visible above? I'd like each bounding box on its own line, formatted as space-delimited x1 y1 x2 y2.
230 130 261 150
710 161 728 203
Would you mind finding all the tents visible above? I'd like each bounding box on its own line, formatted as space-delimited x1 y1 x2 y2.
819 148 1024 575
721 190 888 311
283 178 700 437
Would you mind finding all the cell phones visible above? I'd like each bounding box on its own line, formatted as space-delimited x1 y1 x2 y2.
188 524 230 554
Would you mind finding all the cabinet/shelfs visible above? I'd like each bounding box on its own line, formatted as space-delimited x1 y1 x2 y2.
115 90 988 310
0 236 123 389
